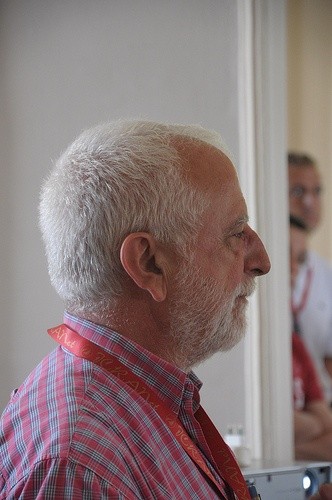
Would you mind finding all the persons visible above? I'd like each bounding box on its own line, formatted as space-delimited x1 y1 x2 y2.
288 150 332 376
288 212 332 460
0 120 271 500
